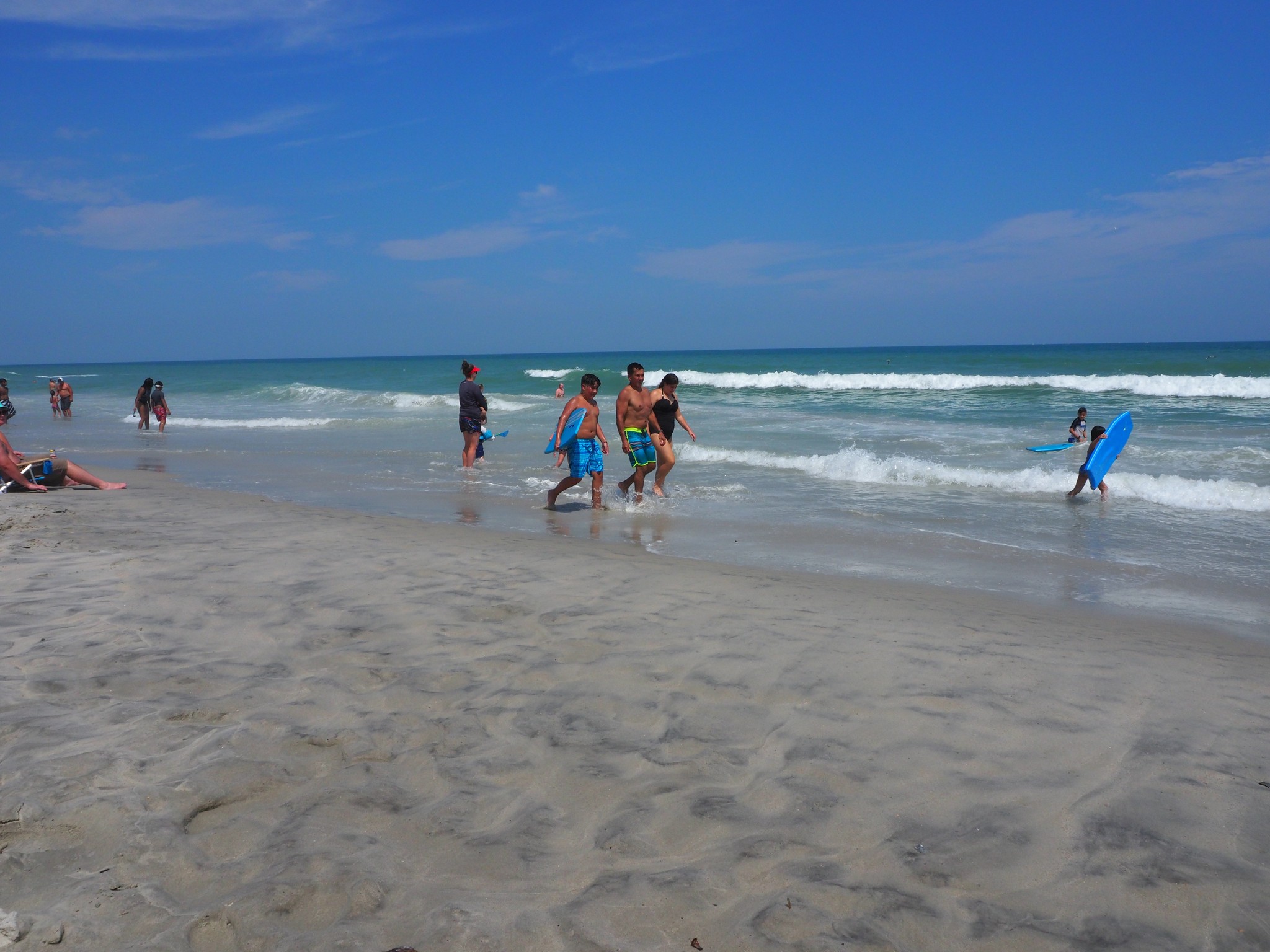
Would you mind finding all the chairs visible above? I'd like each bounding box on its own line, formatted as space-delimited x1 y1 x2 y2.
0 458 49 493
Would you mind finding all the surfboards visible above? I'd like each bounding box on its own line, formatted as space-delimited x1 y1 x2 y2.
1025 441 1075 453
1084 410 1134 491
544 407 588 455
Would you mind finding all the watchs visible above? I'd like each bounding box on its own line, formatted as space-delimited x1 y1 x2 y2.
657 429 664 434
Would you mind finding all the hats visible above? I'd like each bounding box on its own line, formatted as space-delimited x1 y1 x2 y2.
154 381 163 387
465 365 481 376
0 403 9 410
57 378 64 384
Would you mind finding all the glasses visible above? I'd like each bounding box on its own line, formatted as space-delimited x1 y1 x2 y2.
0 409 8 416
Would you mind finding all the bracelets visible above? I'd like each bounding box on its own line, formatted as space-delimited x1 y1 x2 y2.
1078 436 1081 439
602 442 608 445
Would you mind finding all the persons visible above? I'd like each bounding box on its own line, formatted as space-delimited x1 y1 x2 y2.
151 381 171 432
56 377 73 417
478 384 488 424
616 362 666 504
0 378 16 424
1068 426 1119 497
1068 408 1087 442
553 450 569 468
548 374 609 510
49 379 57 391
0 403 25 458
646 374 696 496
459 360 487 467
0 439 127 492
475 414 496 458
555 383 564 398
50 390 61 417
133 378 153 429
